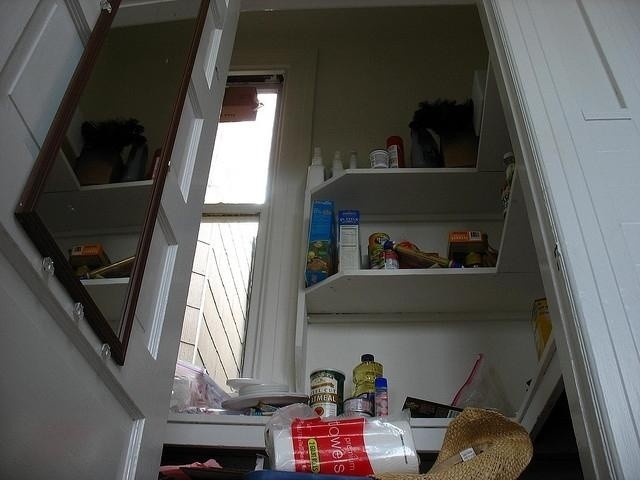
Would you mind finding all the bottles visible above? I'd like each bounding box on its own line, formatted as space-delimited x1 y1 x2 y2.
386 135 405 169
379 240 400 272
351 353 385 401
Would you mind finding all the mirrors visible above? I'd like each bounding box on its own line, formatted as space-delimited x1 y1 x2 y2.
14 0 216 364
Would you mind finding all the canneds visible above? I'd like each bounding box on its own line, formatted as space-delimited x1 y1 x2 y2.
310 369 346 420
343 397 373 417
369 232 390 269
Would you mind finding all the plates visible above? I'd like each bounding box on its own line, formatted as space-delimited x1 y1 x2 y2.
220 377 309 412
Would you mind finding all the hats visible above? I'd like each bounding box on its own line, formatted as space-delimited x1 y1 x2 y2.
368 405 533 479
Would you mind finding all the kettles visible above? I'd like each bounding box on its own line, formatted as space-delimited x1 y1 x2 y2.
374 376 390 419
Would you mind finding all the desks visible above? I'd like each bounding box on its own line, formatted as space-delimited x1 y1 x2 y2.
163 328 561 479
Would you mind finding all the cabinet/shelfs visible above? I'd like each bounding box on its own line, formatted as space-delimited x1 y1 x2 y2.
305 48 542 316
